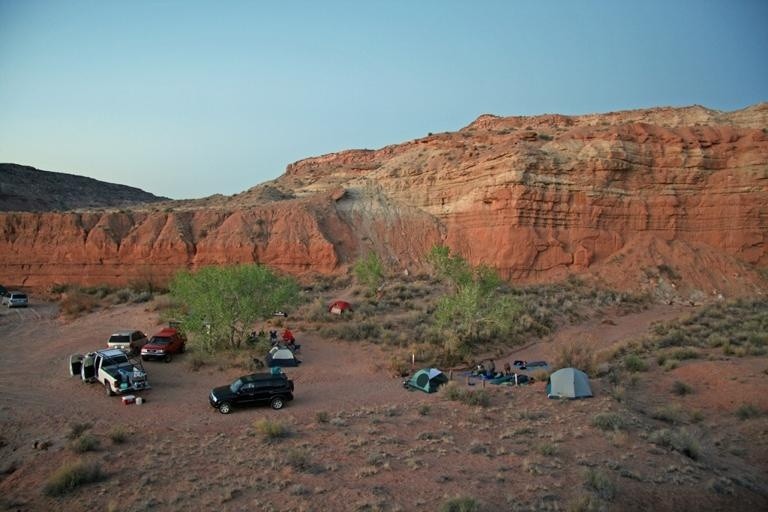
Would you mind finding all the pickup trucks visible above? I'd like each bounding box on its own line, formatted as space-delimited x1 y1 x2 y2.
69 348 152 398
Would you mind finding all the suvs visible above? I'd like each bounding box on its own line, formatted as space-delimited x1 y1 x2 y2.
208 372 295 411
106 330 149 356
140 326 186 362
2 291 27 308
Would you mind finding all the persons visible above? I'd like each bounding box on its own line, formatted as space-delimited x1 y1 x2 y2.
476 358 511 378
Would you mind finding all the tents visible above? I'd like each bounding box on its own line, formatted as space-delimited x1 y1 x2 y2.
265 346 301 367
545 367 594 400
329 300 352 316
408 366 448 393
